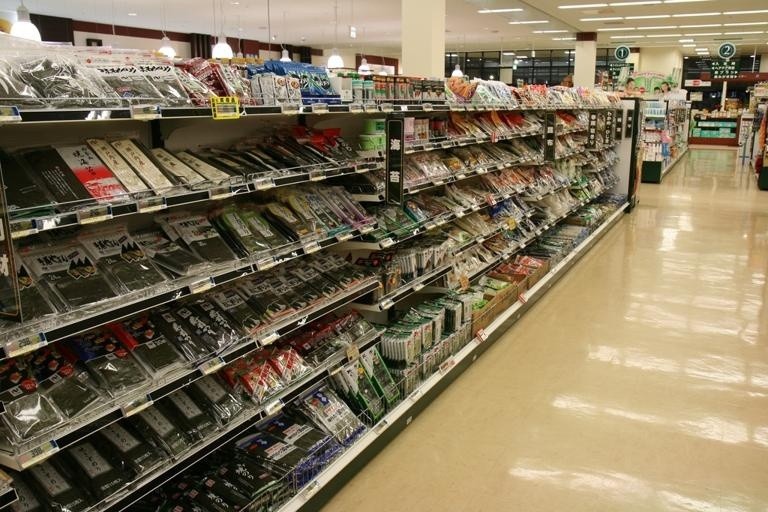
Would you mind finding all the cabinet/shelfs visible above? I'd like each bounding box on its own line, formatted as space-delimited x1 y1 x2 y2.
689 116 741 146
641 99 692 184
0 32 644 512
741 83 768 191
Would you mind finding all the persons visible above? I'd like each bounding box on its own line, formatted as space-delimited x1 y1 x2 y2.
626 77 671 96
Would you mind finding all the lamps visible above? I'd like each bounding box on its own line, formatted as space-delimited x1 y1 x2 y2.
12 0 463 78
